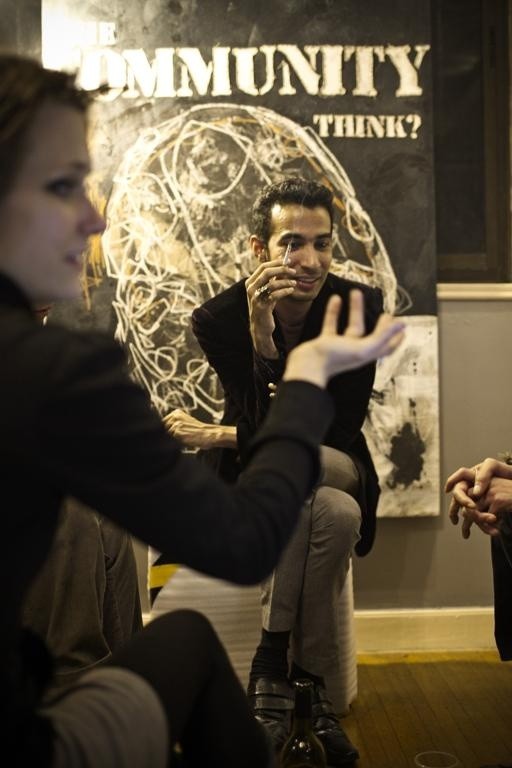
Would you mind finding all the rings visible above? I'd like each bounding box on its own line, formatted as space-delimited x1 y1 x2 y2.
253 283 270 299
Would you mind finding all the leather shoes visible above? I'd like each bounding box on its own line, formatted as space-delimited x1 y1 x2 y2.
310 700 360 764
248 677 296 746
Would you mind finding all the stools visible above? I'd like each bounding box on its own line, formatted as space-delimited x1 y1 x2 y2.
146 539 358 716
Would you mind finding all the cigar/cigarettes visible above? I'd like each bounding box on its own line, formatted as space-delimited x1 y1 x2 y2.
282 243 292 266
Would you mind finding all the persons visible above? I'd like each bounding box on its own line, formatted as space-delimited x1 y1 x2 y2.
439 447 511 667
0 50 409 765
155 174 388 768
